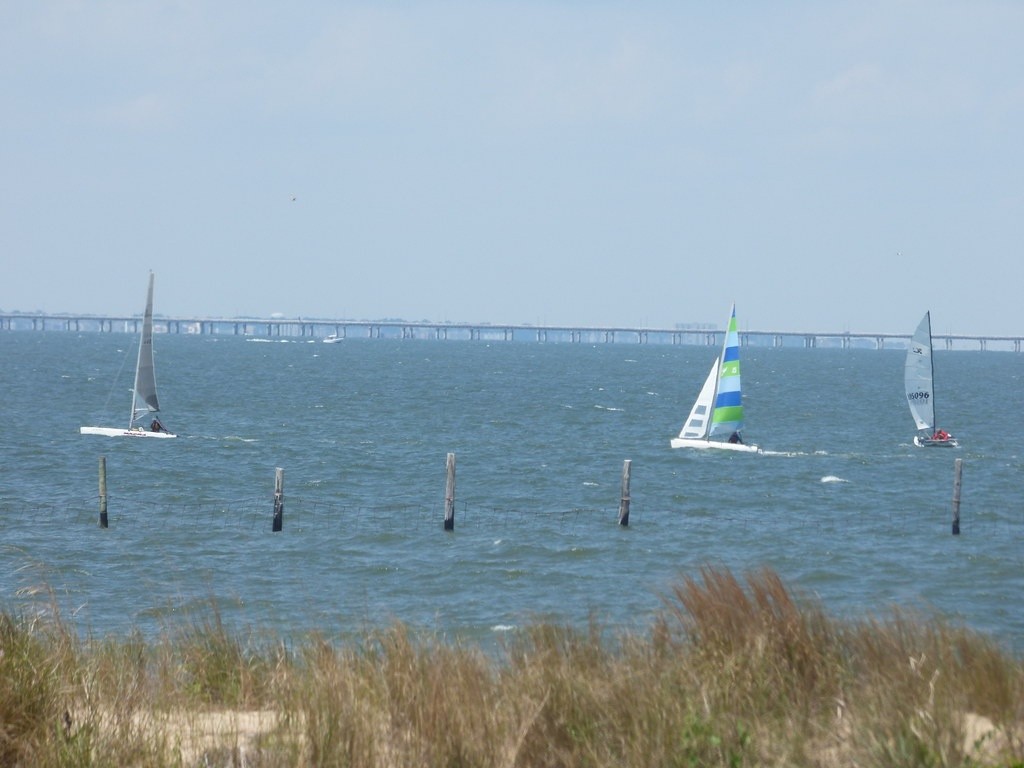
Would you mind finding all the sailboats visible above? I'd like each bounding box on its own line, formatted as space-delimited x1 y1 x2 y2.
669 303 760 454
903 310 959 447
81 270 179 439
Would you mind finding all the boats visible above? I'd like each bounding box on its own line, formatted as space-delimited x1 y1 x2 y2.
323 334 345 344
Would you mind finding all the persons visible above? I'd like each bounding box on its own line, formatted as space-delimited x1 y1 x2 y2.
151 420 160 432
932 429 948 440
729 432 742 444
